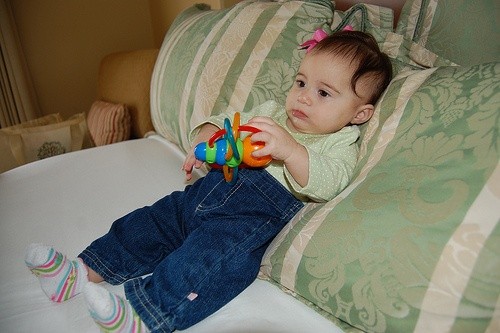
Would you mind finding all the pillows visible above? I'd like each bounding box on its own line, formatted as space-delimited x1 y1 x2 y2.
151 0 500 333
0 100 131 173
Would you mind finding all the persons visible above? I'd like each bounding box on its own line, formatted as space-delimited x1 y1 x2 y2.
26 25 393 333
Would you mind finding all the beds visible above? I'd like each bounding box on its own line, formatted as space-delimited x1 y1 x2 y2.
0 132 346 333
